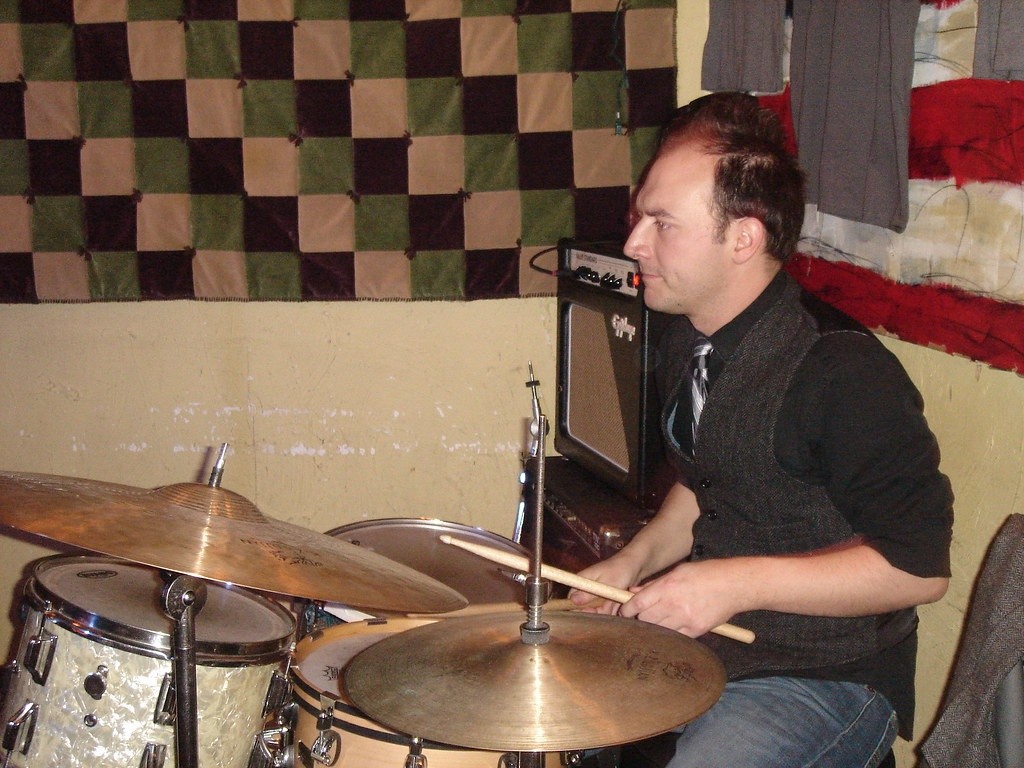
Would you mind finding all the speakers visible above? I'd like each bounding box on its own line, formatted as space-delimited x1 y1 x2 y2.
554 238 698 514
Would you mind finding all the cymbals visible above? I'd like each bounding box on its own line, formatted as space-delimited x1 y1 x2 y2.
339 608 728 753
0 468 472 616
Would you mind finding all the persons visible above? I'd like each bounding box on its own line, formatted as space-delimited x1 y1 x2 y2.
552 91 953 768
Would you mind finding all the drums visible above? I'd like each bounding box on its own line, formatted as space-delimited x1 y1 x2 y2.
316 513 556 623
1 554 300 768
286 612 575 768
